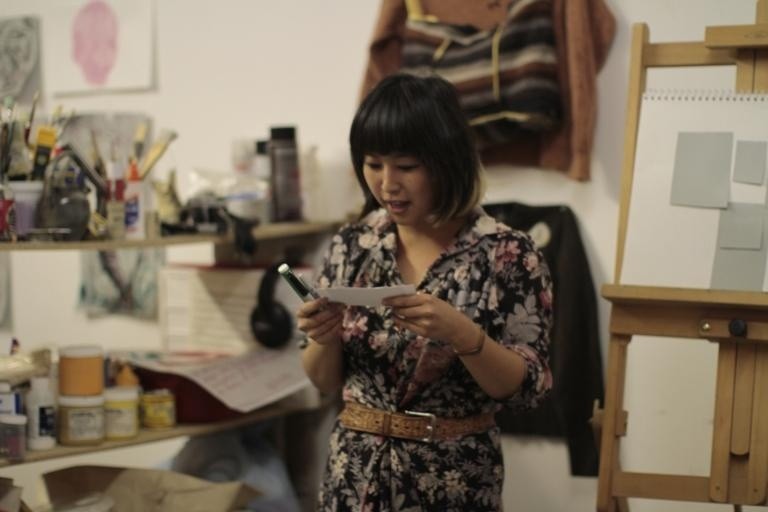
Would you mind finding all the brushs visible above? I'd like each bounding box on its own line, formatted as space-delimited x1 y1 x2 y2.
0 90 177 199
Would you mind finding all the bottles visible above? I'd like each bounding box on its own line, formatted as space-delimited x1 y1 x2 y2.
225 126 302 226
44 185 90 242
104 160 127 238
0 345 177 456
124 165 161 242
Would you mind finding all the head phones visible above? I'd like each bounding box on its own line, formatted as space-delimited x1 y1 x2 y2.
251 264 293 346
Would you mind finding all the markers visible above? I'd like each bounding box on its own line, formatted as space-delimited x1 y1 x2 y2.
277 263 323 312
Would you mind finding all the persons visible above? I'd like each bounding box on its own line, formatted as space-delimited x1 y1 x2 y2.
296 73 554 511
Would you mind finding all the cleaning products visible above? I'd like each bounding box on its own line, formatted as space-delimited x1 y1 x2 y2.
123 163 146 239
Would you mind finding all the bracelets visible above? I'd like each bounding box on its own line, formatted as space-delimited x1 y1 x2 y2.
451 323 485 356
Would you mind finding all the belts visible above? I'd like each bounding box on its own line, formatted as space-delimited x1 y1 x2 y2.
338 403 499 443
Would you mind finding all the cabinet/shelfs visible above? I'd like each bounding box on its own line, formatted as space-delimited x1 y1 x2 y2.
0 218 341 512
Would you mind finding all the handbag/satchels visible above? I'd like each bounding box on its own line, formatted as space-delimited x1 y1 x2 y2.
396 0 564 148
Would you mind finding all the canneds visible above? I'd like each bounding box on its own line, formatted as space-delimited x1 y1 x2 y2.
56 343 178 447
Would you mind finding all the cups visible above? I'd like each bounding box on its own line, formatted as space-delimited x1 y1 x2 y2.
6 180 44 238
